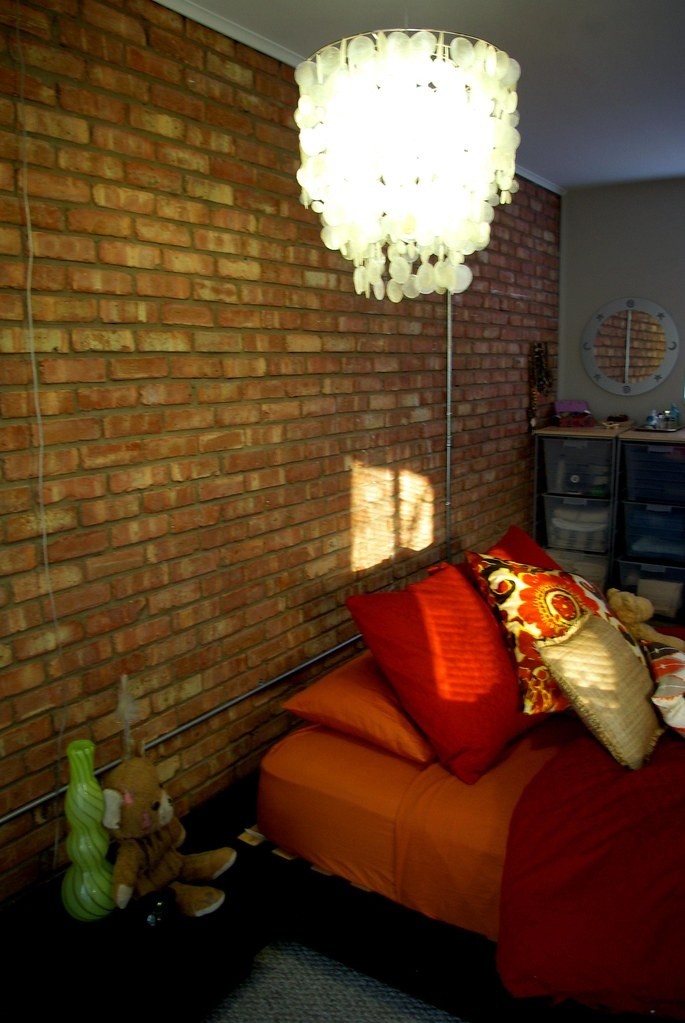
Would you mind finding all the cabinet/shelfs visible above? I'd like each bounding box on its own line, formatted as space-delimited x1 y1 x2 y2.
532 426 685 630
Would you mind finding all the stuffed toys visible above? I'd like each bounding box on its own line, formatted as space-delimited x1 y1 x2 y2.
606 588 685 654
101 756 237 917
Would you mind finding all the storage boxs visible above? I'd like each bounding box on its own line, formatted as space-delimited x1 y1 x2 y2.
542 494 611 553
622 444 685 504
623 500 685 562
542 439 611 498
545 549 609 591
616 558 685 625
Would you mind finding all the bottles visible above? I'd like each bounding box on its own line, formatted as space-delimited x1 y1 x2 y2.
62 738 116 920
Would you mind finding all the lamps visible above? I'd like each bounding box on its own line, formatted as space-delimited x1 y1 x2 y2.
293 13 521 303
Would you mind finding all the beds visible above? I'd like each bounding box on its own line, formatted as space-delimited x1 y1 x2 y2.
256 625 685 1023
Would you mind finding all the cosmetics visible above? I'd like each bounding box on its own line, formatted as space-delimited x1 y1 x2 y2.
645 402 681 429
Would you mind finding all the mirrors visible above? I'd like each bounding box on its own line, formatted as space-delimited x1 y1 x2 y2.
592 310 666 384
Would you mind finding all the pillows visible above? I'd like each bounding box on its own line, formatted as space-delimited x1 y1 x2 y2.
428 525 561 585
343 565 548 784
640 639 685 740
464 550 646 715
279 649 438 766
533 613 663 770
